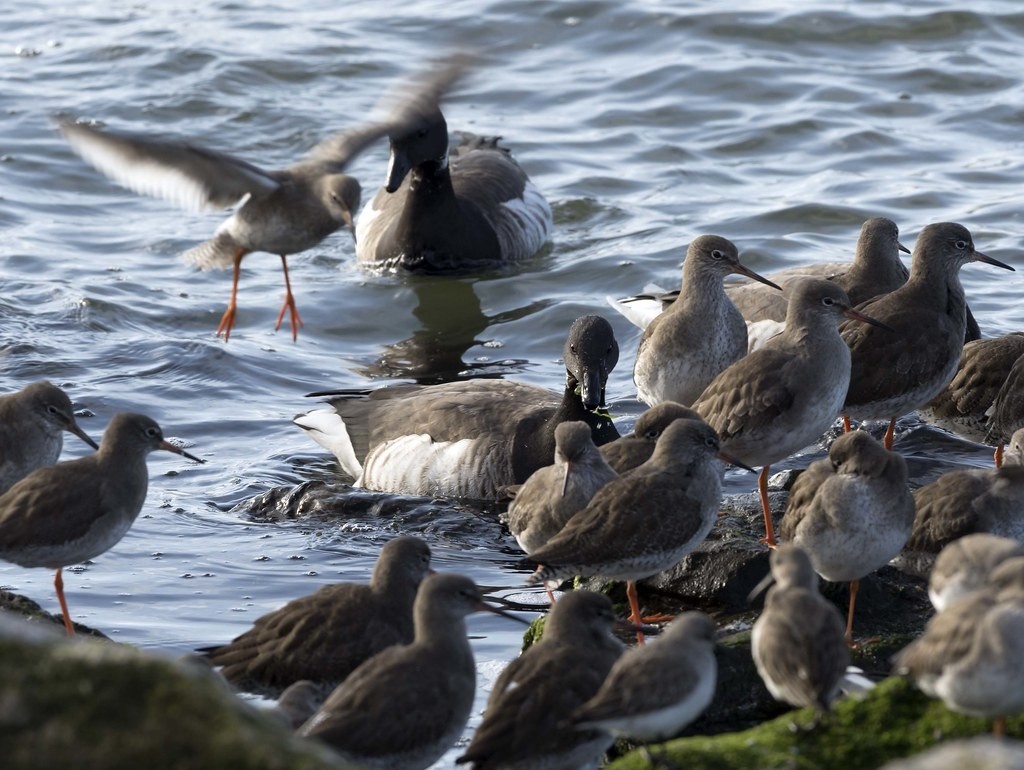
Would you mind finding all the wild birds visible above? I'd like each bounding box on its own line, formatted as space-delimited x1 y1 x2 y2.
0 54 1024 770
